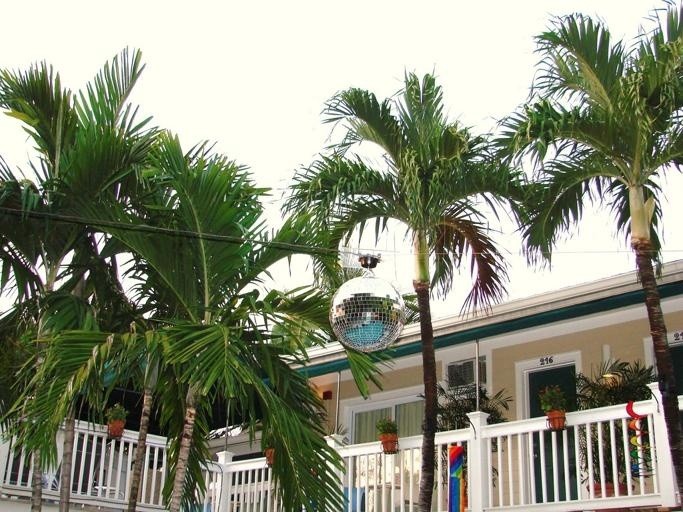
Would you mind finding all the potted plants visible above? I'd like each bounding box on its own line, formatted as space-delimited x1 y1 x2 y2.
538 383 565 431
265 449 276 465
106 403 130 436
565 361 658 512
376 416 398 454
